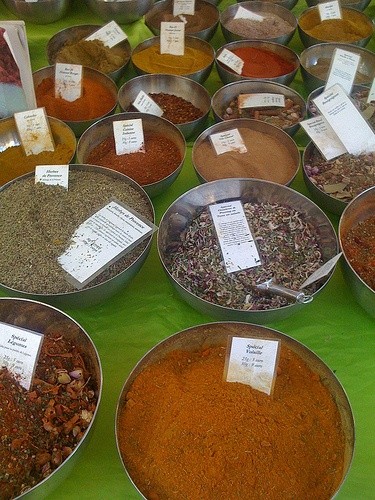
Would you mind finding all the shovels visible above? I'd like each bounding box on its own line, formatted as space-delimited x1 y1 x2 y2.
244 272 314 305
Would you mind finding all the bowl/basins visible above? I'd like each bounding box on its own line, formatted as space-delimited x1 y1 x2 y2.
297 5 374 49
220 2 298 46
215 40 301 87
338 186 375 320
76 113 187 199
211 80 306 137
47 26 131 83
302 139 375 216
192 118 301 188
300 43 375 97
306 84 375 131
144 0 221 42
0 163 155 306
85 0 153 25
2 0 74 25
32 66 118 138
117 73 211 139
157 178 339 322
0 297 104 500
115 320 356 500
131 34 216 85
0 116 77 186
